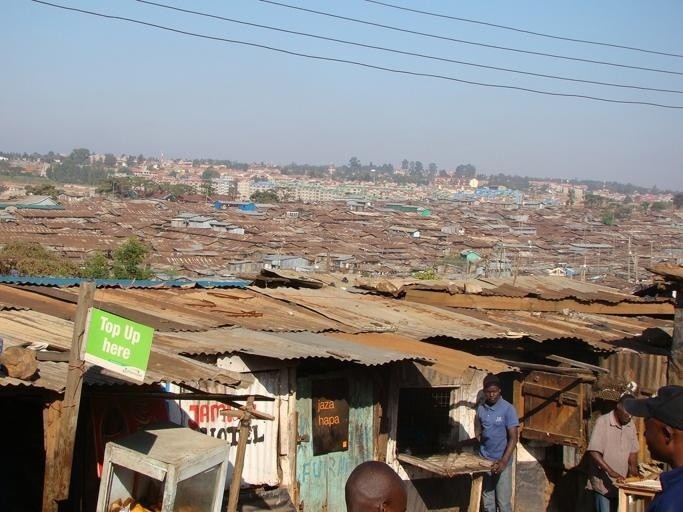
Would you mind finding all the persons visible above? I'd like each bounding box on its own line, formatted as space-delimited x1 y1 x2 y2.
345 460 408 512
623 384 683 512
473 375 520 512
583 394 642 512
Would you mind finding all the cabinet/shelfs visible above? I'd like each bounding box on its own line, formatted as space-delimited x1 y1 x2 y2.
95 418 231 512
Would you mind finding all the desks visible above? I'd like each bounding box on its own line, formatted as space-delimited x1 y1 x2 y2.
612 480 664 512
397 451 499 511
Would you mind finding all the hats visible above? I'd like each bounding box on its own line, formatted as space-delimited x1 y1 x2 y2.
481 375 500 386
623 385 683 430
